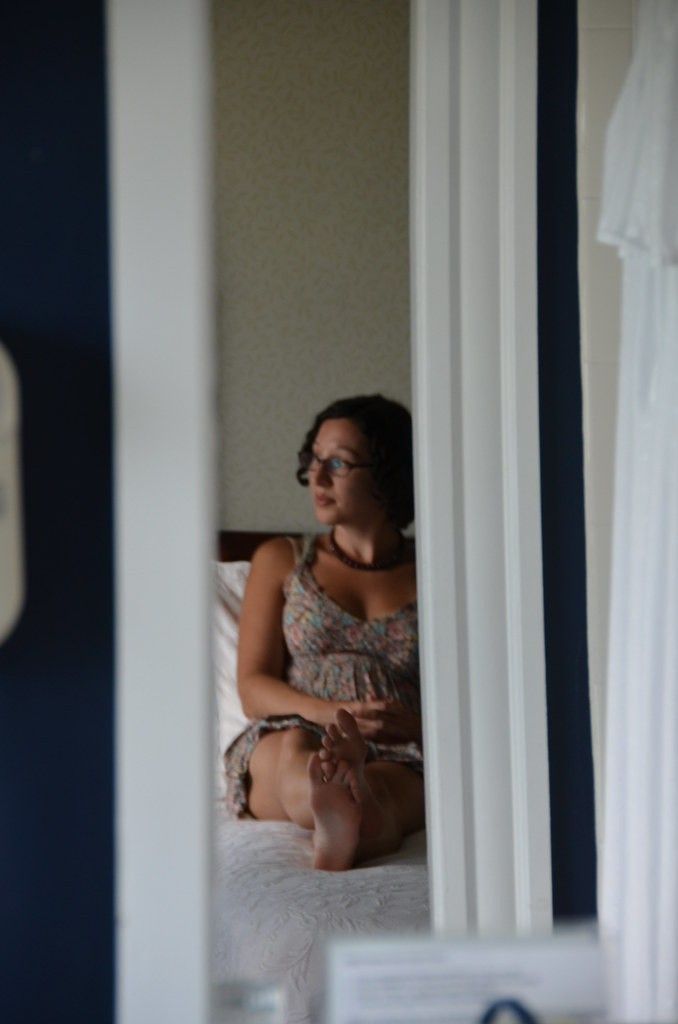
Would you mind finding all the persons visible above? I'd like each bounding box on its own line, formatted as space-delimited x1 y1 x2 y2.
223 393 424 870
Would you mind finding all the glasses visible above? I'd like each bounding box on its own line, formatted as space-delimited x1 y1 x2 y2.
299 452 366 477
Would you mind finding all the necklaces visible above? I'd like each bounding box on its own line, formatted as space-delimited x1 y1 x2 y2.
328 528 406 570
